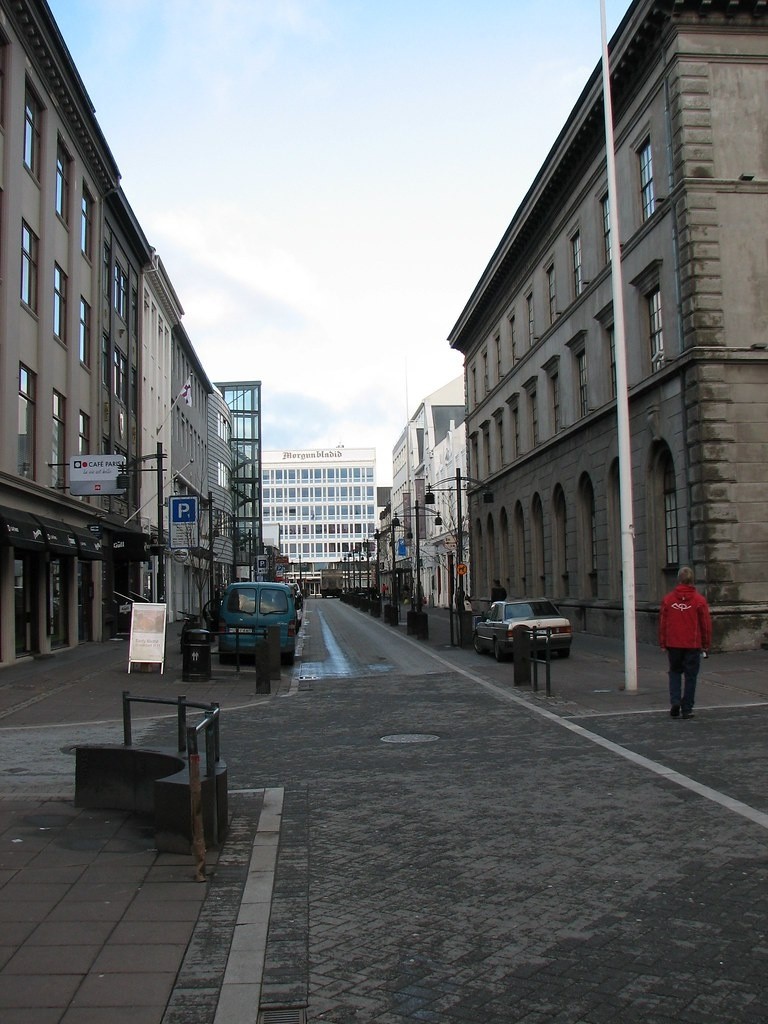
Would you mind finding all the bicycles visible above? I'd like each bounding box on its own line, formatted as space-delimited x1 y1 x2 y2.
177 610 200 654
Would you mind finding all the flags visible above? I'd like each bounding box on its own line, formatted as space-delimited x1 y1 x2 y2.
181 377 193 408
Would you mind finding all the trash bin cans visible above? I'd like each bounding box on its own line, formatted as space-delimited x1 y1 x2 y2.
182 629 212 682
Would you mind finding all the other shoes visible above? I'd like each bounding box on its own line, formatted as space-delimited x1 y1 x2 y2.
670 706 680 717
683 712 694 720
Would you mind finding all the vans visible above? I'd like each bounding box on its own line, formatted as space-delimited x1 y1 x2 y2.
219 583 302 666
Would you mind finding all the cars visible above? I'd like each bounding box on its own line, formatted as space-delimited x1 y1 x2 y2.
473 598 573 662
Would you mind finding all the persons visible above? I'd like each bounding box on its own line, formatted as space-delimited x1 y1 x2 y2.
491 579 507 603
657 567 712 719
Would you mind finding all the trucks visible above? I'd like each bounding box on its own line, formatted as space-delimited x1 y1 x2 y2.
320 569 343 598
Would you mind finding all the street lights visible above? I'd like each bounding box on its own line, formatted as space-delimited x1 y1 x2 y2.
391 499 442 611
373 515 413 606
336 529 392 598
425 468 495 646
